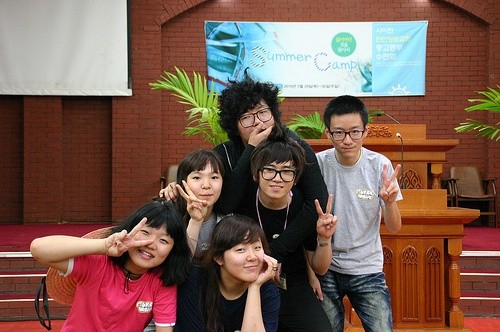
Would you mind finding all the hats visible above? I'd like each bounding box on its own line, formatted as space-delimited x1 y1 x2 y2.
46 227 122 306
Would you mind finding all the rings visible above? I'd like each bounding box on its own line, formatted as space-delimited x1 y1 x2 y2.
272 267 277 271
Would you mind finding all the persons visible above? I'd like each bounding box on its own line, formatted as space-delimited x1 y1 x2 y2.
158 136 338 332
172 216 281 332
162 149 224 263
214 67 332 216
305 95 404 332
30 200 178 332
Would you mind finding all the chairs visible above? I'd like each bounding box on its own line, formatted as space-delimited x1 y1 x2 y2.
441 167 497 227
161 165 179 191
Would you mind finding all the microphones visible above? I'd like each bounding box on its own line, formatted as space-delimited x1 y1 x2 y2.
396 132 406 188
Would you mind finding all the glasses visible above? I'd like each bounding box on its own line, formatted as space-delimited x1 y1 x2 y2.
260 168 297 181
329 130 367 141
237 108 273 128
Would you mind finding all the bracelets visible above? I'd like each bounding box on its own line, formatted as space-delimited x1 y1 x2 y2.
316 237 331 247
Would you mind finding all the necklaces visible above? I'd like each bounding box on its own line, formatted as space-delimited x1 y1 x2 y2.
123 266 143 294
256 186 293 239
